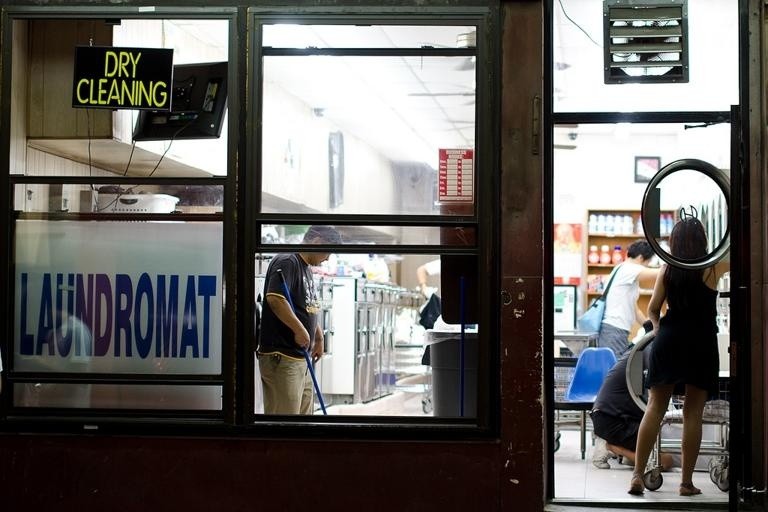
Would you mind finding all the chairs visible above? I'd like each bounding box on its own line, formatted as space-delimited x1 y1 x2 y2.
567 346 618 399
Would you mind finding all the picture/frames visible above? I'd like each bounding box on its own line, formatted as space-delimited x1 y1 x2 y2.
634 155 662 184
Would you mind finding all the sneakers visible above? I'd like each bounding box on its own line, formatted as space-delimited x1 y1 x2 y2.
591 436 614 469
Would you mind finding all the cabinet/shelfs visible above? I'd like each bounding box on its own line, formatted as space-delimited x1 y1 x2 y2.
262 96 394 218
312 274 333 411
586 208 676 325
28 17 230 179
349 278 394 404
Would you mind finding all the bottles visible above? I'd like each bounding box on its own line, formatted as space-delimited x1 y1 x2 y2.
589 213 674 266
714 272 730 334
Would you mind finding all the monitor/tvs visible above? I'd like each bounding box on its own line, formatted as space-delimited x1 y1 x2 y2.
132 62 228 140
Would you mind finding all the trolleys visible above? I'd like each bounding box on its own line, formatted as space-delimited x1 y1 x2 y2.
415 287 434 414
626 316 729 493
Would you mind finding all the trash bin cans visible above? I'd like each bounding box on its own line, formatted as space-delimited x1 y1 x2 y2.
429 330 478 417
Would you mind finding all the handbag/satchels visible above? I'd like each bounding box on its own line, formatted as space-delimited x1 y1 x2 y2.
577 297 605 333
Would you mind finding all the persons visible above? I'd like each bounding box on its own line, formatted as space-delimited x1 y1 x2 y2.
590 352 650 468
626 215 729 497
574 237 663 360
416 257 440 299
256 220 343 416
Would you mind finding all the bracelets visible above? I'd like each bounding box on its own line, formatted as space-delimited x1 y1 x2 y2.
420 282 428 288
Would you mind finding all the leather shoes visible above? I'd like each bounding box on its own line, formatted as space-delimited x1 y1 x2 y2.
680 481 700 495
628 475 645 495
